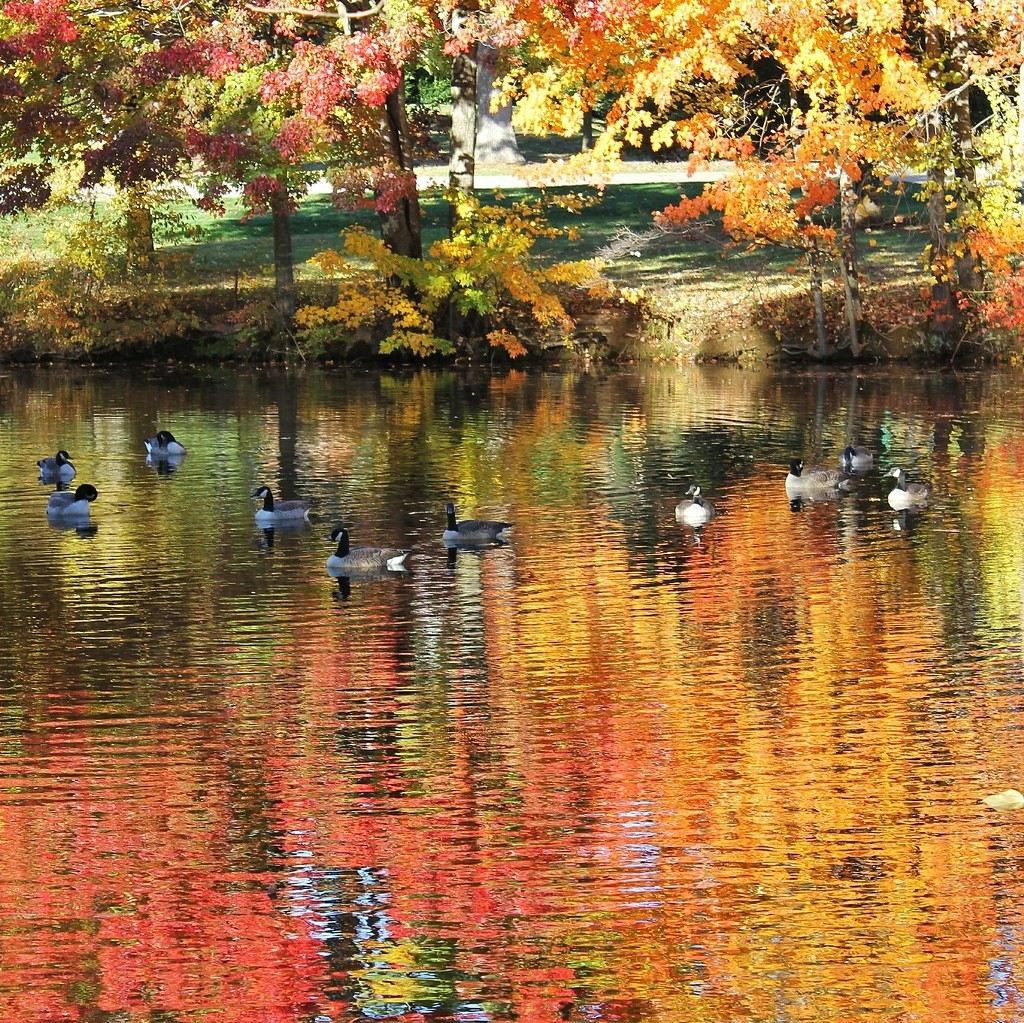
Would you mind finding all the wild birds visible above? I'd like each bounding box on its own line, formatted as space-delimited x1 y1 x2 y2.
883 466 932 515
785 446 875 504
141 429 189 459
248 484 519 579
35 449 98 519
674 483 720 534
